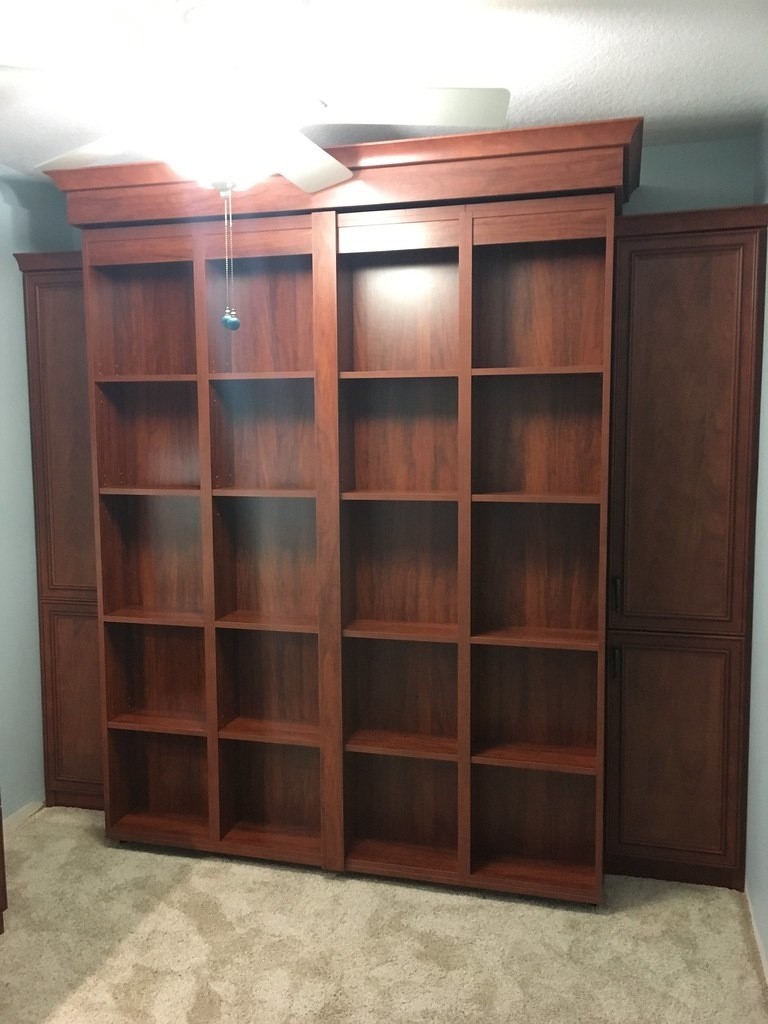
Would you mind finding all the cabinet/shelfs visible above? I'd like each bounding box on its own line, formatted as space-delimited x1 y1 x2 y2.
41 118 650 908
12 250 110 811
598 204 768 893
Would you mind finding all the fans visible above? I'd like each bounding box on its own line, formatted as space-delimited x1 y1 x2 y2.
41 78 510 196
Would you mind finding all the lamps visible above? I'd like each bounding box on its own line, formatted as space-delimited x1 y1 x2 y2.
149 117 295 330
